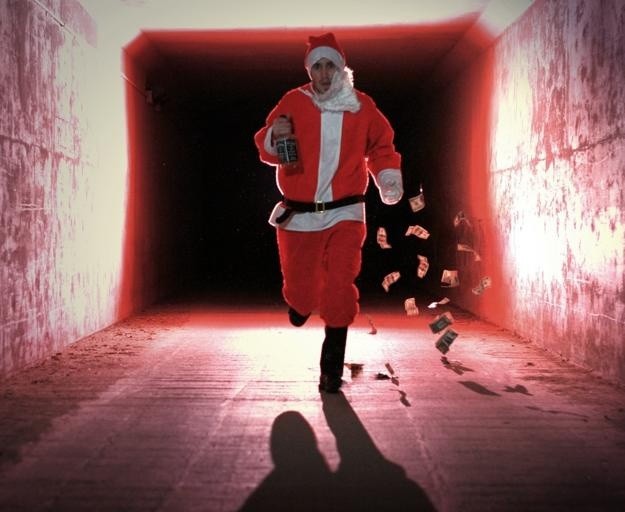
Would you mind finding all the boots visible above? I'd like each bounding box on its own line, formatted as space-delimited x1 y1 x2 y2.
318 327 345 392
287 306 310 327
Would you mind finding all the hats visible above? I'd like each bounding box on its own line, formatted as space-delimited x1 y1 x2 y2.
305 33 346 77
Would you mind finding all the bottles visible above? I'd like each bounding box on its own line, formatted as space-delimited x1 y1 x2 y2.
275 114 300 169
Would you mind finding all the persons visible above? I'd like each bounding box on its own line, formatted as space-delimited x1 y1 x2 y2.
253 32 406 395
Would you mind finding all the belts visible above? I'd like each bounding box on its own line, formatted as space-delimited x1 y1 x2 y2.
277 196 361 225
